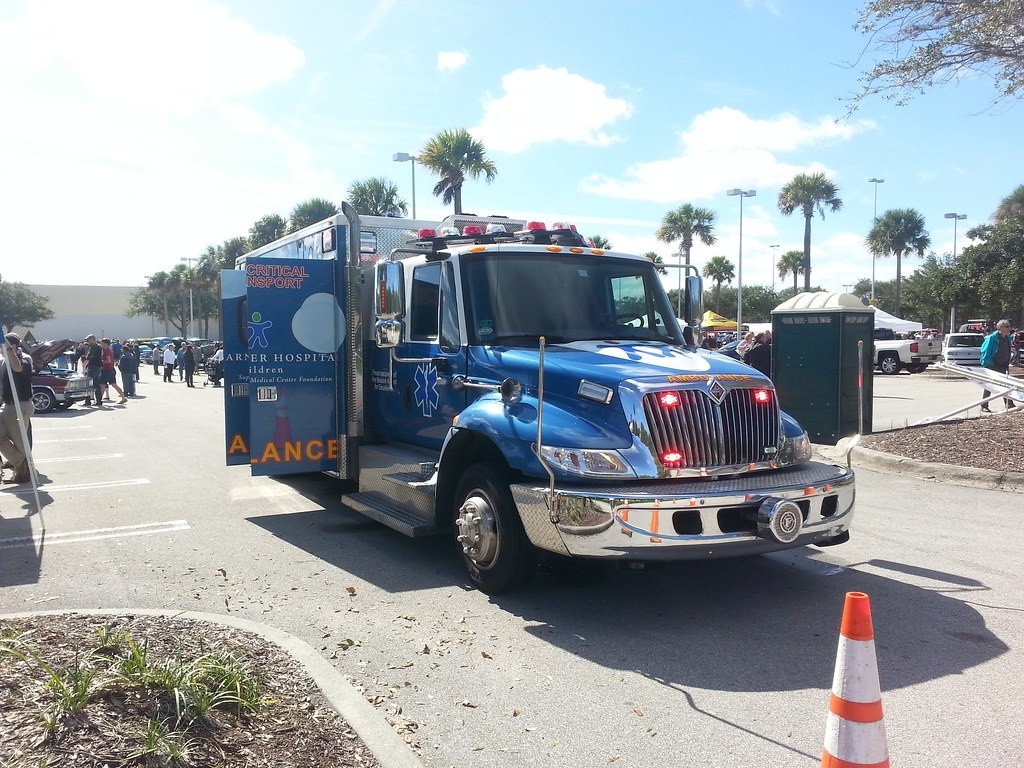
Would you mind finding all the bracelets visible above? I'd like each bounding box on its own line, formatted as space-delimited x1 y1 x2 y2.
6 346 13 351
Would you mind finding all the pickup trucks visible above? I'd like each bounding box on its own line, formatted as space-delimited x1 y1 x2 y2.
873 329 940 376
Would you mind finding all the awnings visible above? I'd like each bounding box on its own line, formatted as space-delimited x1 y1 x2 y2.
868 305 923 333
624 310 749 333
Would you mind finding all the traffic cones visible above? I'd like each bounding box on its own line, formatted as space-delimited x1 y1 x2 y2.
817 589 894 768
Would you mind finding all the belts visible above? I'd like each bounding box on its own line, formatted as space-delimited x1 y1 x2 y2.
5 398 31 401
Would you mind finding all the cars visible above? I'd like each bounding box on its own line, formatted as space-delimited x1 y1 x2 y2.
117 337 230 380
943 334 988 365
13 340 96 412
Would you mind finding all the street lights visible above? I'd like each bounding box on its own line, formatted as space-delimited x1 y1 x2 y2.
391 150 429 218
181 256 200 340
869 176 885 306
727 187 757 344
945 211 967 337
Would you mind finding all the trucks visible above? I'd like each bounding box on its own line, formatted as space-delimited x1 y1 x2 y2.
219 203 859 599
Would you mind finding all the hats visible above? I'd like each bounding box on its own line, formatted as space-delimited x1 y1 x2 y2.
84 334 96 341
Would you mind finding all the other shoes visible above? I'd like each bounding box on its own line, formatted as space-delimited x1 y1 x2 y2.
1 460 14 470
91 401 102 406
3 476 31 484
981 408 991 412
187 383 195 387
78 401 91 407
103 397 110 400
117 398 128 404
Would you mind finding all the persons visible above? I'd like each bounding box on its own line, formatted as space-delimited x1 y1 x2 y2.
894 331 915 340
700 330 771 378
979 318 1021 412
31 334 140 407
152 341 224 387
0 332 35 483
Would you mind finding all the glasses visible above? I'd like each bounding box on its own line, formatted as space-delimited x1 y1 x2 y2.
1001 324 1012 329
